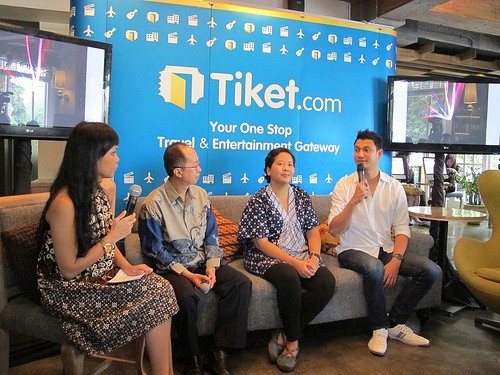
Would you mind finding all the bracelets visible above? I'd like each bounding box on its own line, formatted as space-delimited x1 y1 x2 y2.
311 252 322 263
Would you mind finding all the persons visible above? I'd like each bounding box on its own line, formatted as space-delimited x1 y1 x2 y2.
328 128 437 355
138 143 252 375
398 153 425 206
36 122 179 375
444 154 460 192
237 148 336 371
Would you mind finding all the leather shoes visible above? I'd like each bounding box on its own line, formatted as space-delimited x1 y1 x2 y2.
184 356 203 375
206 348 232 375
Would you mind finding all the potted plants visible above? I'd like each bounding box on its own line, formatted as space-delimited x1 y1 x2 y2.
459 176 486 225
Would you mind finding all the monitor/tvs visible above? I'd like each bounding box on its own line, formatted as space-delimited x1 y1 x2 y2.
423 157 449 180
0 25 112 141
386 76 500 155
391 157 406 179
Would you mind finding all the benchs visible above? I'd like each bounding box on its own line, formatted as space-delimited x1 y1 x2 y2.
123 184 443 338
430 191 463 209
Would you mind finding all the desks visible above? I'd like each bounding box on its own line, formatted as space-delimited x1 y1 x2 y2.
409 207 487 310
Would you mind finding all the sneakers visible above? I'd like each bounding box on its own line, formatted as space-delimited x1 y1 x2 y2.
368 328 388 355
387 324 429 346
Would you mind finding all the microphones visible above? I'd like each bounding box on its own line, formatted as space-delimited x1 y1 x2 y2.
126 184 142 218
200 279 210 293
357 162 364 183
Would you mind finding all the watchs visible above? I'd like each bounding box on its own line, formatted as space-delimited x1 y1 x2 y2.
100 240 113 252
393 254 403 260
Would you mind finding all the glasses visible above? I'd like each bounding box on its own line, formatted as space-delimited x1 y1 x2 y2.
170 162 200 173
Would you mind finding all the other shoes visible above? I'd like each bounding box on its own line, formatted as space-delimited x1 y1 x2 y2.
268 330 287 362
277 343 300 371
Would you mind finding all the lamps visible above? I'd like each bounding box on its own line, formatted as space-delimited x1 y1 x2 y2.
54 71 67 100
464 83 480 116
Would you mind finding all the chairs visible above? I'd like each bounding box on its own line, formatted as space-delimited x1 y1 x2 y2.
0 178 147 375
454 170 500 329
